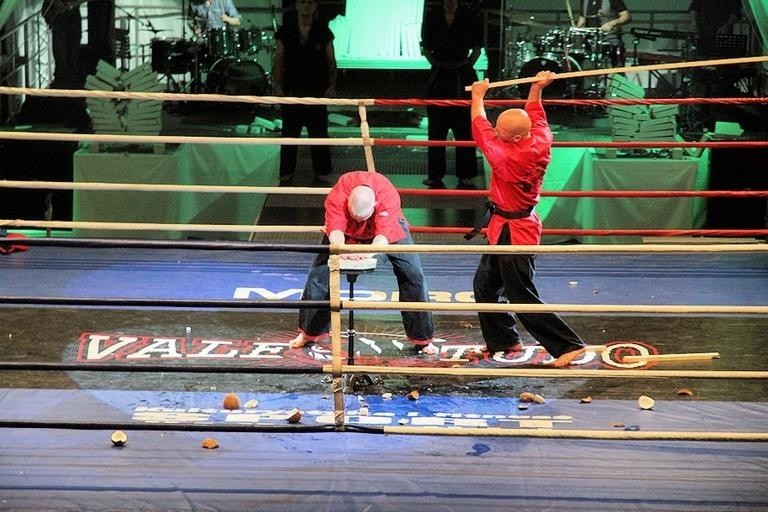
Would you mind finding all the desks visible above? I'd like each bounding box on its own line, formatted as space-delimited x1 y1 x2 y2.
582 145 711 244
73 144 188 239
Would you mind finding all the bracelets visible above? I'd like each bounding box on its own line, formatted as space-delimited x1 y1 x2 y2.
723 21 733 29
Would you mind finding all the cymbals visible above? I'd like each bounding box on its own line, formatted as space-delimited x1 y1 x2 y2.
512 19 550 28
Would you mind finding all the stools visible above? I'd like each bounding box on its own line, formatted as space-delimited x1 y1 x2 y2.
340 258 378 365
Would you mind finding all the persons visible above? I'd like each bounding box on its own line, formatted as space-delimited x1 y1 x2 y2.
686 0 745 57
287 168 440 355
572 0 631 76
418 0 485 186
41 1 83 84
270 1 339 188
464 69 586 371
187 1 242 73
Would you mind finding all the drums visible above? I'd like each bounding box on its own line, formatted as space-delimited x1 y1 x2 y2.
208 26 237 60
207 56 268 94
241 27 263 51
566 32 592 56
519 54 569 99
534 33 563 52
152 38 189 74
